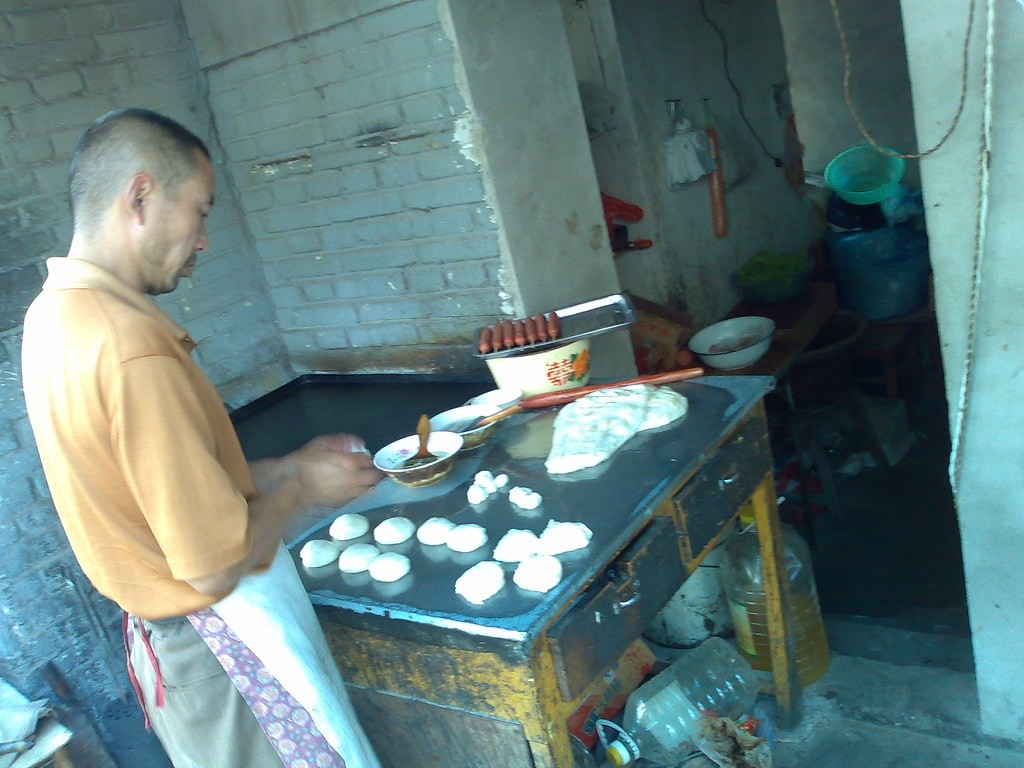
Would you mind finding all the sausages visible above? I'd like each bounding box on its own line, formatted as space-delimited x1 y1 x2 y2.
478 312 559 352
676 343 694 367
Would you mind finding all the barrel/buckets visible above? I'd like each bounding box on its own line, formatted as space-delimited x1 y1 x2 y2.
638 519 736 646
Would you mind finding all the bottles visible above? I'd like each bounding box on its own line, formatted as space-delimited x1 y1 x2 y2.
722 497 831 694
596 637 758 765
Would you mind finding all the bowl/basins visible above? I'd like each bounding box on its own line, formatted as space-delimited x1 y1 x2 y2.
428 404 503 451
733 251 816 303
485 335 591 401
373 431 464 489
688 315 776 371
471 387 524 418
824 145 906 206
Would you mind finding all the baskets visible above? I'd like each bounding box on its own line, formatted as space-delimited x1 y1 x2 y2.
825 144 904 206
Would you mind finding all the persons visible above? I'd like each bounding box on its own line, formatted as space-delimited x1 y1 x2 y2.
21 110 385 768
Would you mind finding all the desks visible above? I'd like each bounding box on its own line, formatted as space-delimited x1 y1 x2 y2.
285 374 800 768
678 285 843 412
829 318 918 399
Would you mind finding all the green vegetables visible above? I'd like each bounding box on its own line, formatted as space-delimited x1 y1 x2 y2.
735 247 810 289
